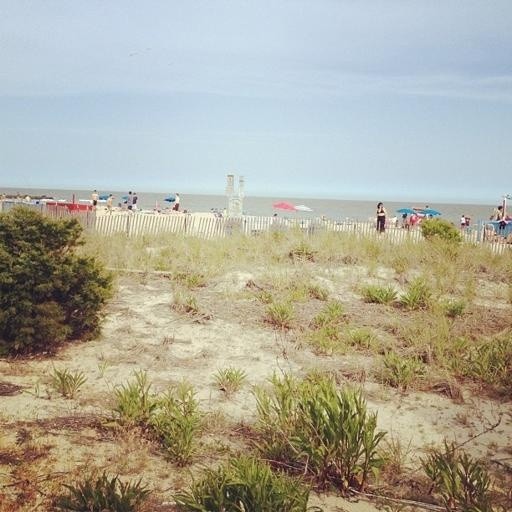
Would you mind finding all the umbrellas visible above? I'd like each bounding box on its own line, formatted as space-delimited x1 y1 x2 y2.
397 206 416 216
274 201 314 213
419 209 444 218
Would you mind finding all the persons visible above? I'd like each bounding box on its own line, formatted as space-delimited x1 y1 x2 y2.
460 215 466 229
493 206 503 220
377 203 386 232
173 193 180 210
105 193 113 215
127 191 133 209
92 190 97 206
132 193 138 209
401 214 417 226
465 218 470 226
272 212 279 217
2 192 32 202
211 208 227 217
